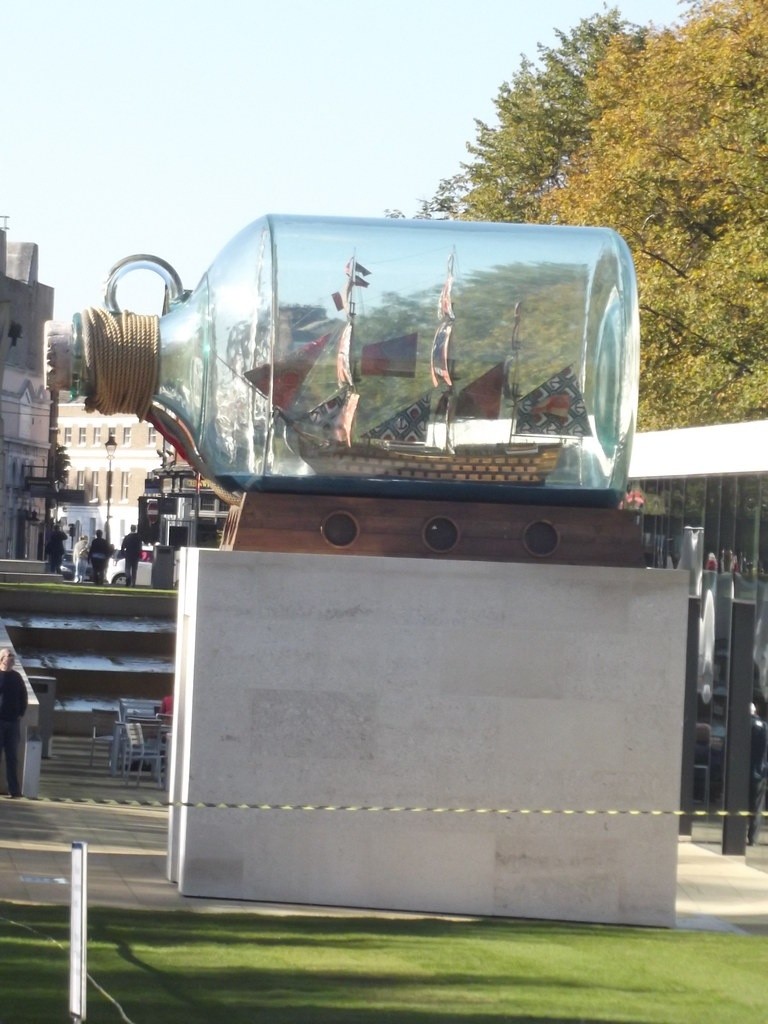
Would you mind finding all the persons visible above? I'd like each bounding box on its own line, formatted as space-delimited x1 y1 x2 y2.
159 678 174 771
0 649 28 798
73 536 90 583
121 525 142 586
88 529 108 586
706 553 718 571
748 702 768 846
48 525 67 574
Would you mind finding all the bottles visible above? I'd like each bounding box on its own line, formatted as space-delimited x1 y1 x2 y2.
47 214 641 509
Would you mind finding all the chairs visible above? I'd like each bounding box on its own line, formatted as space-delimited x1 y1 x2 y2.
125 722 163 788
89 726 114 768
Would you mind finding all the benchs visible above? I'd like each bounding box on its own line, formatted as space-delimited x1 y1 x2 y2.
118 698 163 721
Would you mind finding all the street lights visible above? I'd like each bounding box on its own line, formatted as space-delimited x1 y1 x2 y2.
103 433 118 546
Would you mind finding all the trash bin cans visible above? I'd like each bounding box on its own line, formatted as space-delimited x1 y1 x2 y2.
151 545 174 590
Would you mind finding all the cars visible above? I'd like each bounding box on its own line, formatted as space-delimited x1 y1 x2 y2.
58 551 77 578
103 544 155 589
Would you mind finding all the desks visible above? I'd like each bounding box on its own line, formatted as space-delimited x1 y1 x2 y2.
110 721 171 776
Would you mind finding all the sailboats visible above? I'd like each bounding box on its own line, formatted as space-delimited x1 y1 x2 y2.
231 253 595 487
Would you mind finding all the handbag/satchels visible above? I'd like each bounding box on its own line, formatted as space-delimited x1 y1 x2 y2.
44 539 54 553
79 543 90 559
